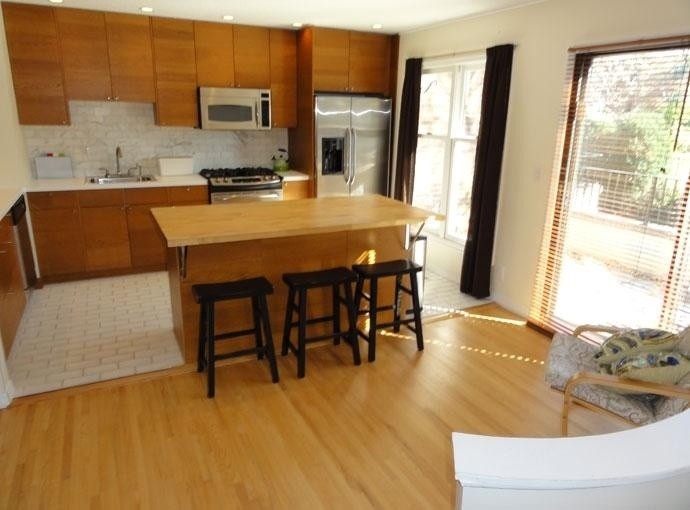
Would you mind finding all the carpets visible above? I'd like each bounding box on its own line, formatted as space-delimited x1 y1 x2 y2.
6 268 495 400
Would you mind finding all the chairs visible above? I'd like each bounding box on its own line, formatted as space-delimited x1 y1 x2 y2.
544 324 690 435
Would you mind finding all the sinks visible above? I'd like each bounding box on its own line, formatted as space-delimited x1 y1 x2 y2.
83 175 158 185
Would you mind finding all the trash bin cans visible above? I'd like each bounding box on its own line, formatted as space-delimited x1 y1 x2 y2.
406 236 427 314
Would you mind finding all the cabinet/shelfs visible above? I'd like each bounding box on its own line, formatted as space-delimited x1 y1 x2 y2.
1 1 71 126
0 215 28 363
298 24 391 94
270 28 298 129
125 185 208 275
151 17 199 128
26 190 132 285
283 181 310 201
193 20 271 92
55 7 155 103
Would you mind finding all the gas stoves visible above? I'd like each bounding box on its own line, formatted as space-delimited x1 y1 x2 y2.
199 167 283 188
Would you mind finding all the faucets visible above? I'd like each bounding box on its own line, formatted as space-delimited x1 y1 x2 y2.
115 146 123 175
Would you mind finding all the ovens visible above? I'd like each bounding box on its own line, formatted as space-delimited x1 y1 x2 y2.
209 186 285 204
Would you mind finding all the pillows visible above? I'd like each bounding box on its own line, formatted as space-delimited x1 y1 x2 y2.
592 327 684 363
597 351 690 385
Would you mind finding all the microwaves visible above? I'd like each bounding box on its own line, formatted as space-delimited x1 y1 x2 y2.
199 85 273 132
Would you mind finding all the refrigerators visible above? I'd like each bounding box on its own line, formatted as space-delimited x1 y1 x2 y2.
313 93 391 198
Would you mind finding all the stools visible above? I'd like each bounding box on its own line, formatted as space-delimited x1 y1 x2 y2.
282 266 361 378
191 276 280 397
351 259 423 362
